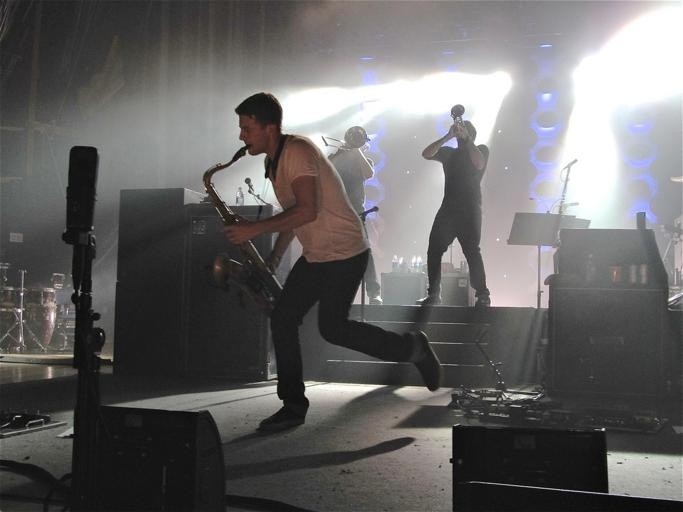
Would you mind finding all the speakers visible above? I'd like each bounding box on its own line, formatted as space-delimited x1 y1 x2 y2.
173 203 291 380
115 187 185 282
112 282 184 390
548 287 669 399
451 425 609 512
99 404 227 512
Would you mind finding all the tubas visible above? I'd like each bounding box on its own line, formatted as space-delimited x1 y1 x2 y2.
323 126 371 150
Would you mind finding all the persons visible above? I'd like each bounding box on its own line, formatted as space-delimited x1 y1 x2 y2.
222 93 443 431
328 133 382 304
416 121 491 307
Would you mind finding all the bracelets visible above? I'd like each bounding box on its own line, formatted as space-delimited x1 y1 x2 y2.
463 136 473 144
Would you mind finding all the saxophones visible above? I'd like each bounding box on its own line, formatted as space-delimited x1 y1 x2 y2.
203 145 284 318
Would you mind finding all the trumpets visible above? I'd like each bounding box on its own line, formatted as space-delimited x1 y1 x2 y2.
451 105 465 135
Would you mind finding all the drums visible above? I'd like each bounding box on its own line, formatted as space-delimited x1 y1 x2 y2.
0 286 27 308
29 288 56 306
24 303 56 351
0 308 24 353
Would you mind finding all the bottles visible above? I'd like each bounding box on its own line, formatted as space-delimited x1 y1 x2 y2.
235 187 244 206
392 255 422 273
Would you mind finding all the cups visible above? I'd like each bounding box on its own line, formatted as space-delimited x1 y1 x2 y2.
608 266 622 284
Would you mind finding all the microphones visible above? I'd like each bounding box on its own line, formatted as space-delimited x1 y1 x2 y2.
244 177 252 189
360 206 379 218
562 158 578 168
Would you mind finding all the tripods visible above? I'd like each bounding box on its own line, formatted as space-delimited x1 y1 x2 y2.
0 269 48 354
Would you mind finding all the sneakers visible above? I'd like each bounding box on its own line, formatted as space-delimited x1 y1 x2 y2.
260 406 306 431
416 296 441 305
406 330 441 391
476 297 490 310
369 295 383 305
